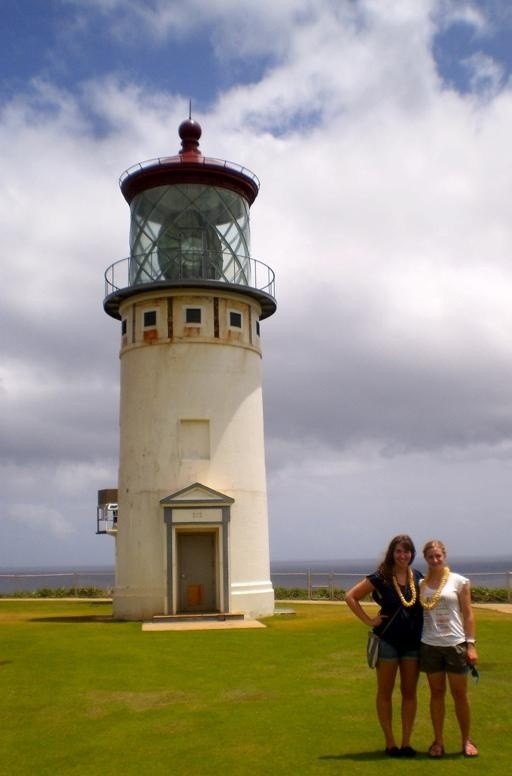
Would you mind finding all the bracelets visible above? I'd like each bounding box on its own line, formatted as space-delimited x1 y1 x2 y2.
465 638 477 645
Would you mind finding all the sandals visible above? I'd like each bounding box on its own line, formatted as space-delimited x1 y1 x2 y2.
428 741 445 758
384 746 417 757
463 742 478 758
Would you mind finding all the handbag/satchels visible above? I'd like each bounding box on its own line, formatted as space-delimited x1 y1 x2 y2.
366 631 381 669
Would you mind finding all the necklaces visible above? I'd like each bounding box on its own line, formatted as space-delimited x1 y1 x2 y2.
419 567 449 609
391 566 416 607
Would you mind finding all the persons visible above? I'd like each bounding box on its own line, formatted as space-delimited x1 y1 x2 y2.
344 534 425 758
415 540 483 759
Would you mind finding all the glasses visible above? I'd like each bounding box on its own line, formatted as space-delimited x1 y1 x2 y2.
467 663 482 687
